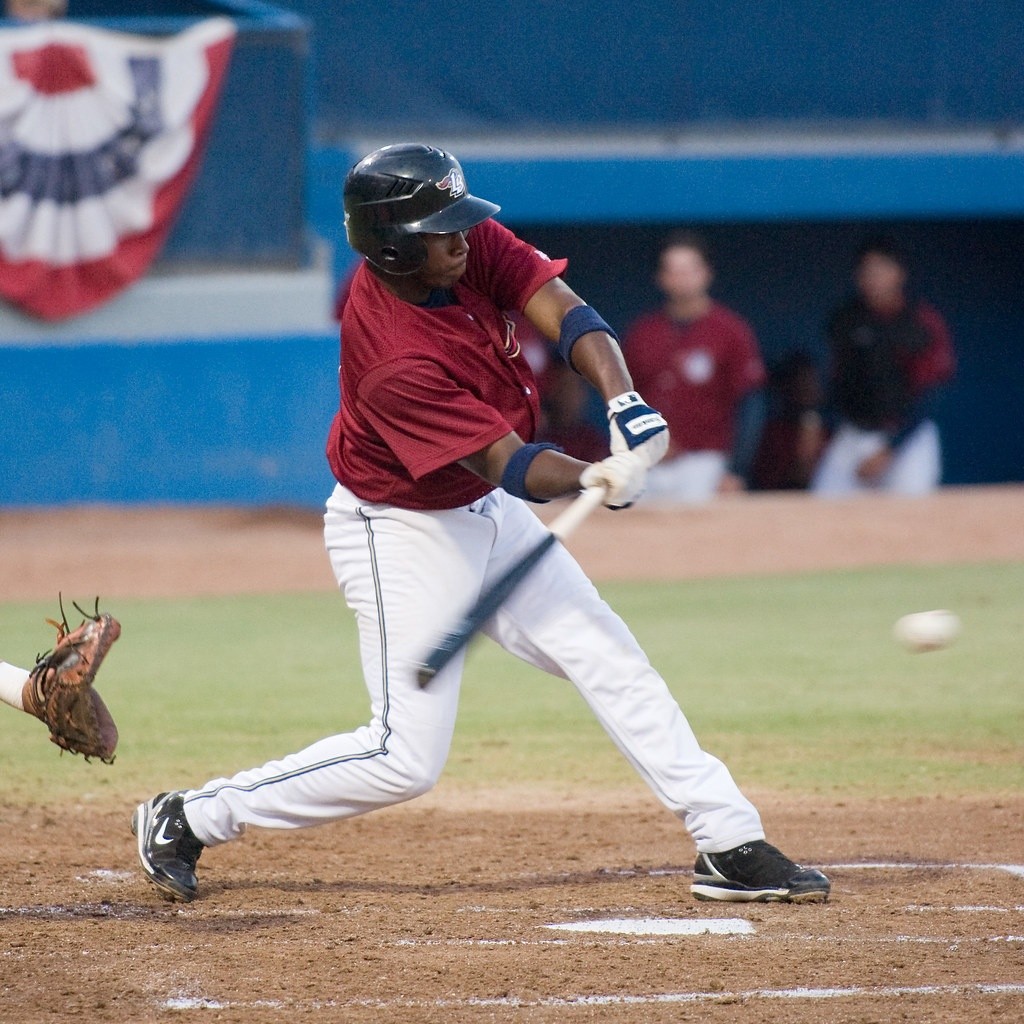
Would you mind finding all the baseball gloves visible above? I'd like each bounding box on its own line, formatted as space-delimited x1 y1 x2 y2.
16 603 135 777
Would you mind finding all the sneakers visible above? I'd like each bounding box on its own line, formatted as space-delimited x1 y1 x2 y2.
130 791 200 902
692 842 831 907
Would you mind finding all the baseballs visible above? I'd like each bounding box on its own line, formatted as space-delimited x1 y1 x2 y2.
889 603 967 658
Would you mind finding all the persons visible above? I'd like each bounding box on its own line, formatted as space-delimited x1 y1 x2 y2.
508 229 959 501
0 598 120 765
131 142 832 902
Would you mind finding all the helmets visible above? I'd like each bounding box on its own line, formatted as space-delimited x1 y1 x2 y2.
345 143 497 275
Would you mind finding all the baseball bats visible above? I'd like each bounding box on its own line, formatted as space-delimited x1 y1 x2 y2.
397 476 612 696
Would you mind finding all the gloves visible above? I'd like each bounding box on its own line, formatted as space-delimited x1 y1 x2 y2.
607 392 669 463
580 452 647 511
